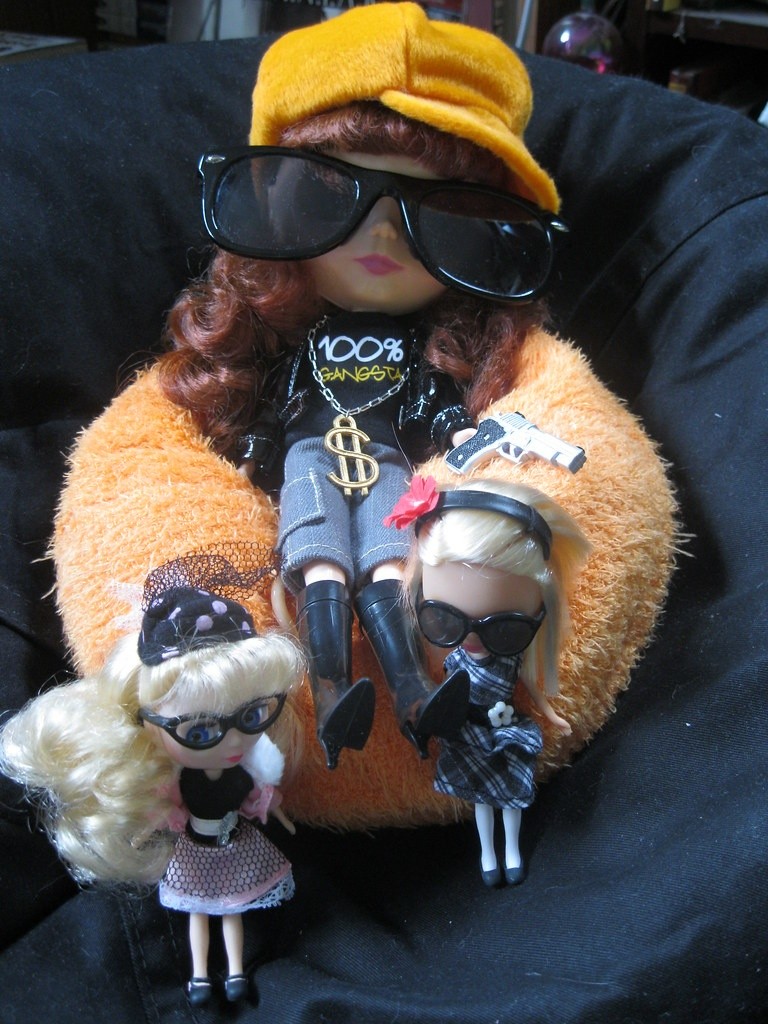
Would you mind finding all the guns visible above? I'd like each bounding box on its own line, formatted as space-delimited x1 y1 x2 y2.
444 409 588 475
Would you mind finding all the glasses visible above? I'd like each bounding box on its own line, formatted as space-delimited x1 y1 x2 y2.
197 145 572 302
413 591 546 658
134 692 290 750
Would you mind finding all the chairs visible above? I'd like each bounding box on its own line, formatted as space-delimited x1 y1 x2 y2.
0 42 768 1024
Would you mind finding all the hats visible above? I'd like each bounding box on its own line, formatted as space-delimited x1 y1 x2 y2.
249 2 561 218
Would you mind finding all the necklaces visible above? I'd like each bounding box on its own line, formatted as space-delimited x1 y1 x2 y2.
307 314 415 496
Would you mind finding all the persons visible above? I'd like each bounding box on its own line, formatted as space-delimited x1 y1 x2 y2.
384 450 596 889
0 542 312 1007
156 0 571 770
